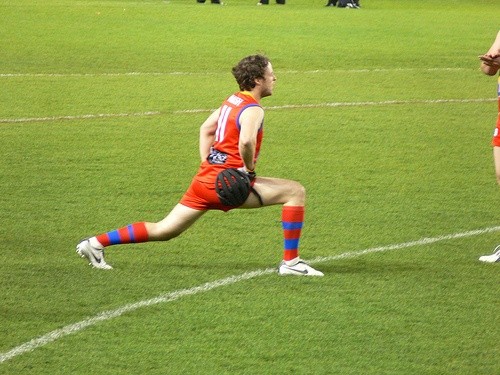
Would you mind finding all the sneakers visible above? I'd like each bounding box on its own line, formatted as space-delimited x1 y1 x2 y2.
479 244 500 263
278 260 324 277
76 239 113 270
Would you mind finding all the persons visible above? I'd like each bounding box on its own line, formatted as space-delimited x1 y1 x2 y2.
476 30 500 263
76 55 324 277
256 0 286 6
196 0 221 4
324 0 359 9
